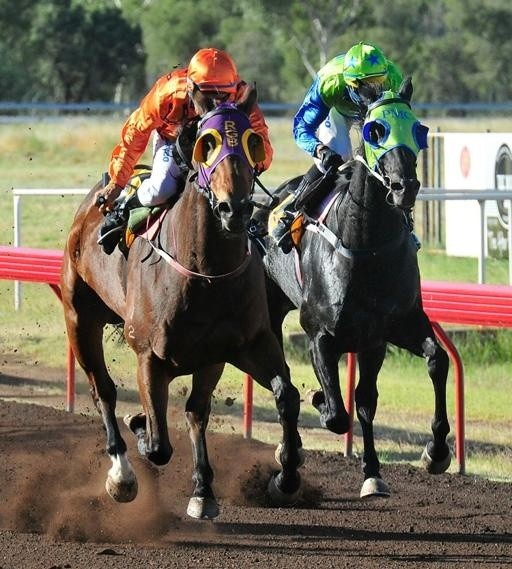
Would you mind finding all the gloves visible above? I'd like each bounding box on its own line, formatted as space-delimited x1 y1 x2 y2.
93 183 122 212
319 146 344 175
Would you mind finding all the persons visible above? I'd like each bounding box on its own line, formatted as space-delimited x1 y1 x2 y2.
94 47 272 256
272 40 421 254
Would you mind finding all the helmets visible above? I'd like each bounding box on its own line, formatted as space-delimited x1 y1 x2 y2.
186 48 239 102
344 41 389 92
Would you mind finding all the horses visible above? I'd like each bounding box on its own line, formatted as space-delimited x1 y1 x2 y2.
59 77 301 520
249 75 450 501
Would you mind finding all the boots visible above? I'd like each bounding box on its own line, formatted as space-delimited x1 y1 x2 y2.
272 176 311 246
100 189 143 255
